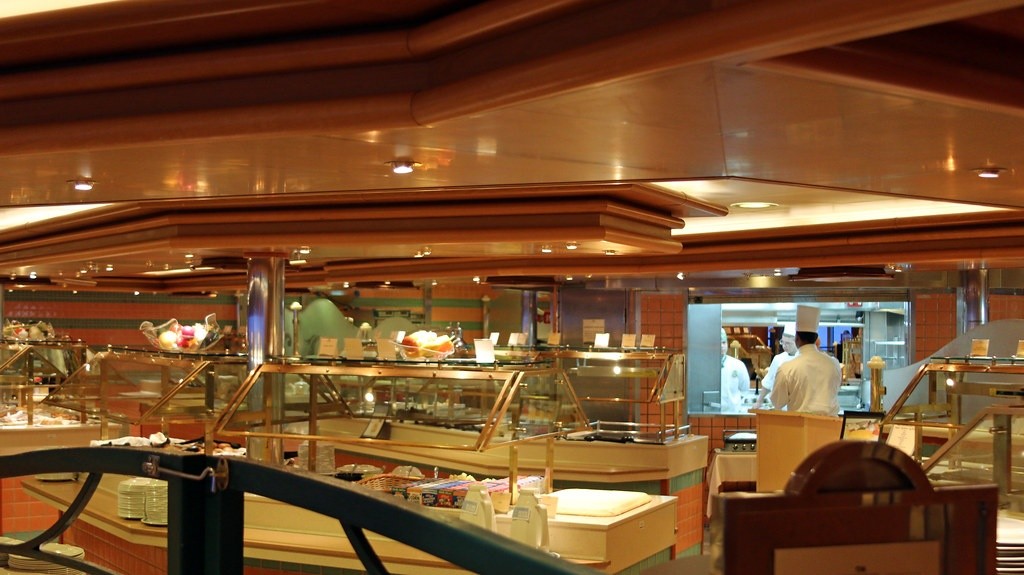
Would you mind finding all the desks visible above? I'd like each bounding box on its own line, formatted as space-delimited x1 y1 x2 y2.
710 449 756 493
496 495 679 575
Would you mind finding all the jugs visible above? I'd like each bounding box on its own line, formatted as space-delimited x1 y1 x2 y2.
509 488 550 553
459 485 497 532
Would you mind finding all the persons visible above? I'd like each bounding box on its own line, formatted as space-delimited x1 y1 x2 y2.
753 328 801 411
771 324 840 416
721 328 750 408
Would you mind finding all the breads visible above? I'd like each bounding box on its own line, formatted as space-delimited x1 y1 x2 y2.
403 330 453 357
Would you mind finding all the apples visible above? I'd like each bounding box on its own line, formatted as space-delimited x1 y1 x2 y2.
169 323 195 349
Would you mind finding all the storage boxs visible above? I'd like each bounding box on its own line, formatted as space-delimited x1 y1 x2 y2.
391 478 545 510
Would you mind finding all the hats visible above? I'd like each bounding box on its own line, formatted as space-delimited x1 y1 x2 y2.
783 323 796 336
797 304 820 332
721 328 727 342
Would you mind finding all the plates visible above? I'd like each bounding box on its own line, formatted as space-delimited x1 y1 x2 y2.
116 479 168 525
996 518 1024 575
0 537 85 575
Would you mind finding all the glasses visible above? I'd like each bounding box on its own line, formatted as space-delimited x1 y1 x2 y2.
779 339 794 346
